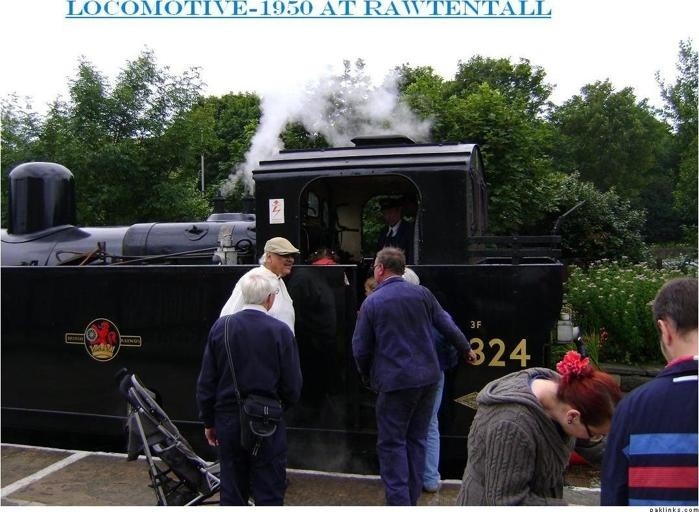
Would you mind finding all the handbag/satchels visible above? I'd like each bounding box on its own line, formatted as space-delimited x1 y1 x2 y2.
240 394 283 459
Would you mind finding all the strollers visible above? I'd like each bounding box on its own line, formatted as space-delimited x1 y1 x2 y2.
119 372 261 506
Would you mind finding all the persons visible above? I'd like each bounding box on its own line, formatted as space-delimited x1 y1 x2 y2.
219 236 300 339
455 349 624 506
401 268 445 494
376 198 414 255
195 273 304 506
364 276 377 296
307 246 341 265
600 278 698 506
352 246 478 506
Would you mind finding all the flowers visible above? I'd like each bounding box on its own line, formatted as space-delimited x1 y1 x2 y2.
556 349 591 383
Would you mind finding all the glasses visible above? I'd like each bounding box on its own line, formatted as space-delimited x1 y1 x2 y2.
572 400 605 443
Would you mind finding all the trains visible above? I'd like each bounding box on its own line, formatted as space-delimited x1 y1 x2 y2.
0 128 608 485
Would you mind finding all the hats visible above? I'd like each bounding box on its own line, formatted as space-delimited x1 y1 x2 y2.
264 236 301 255
